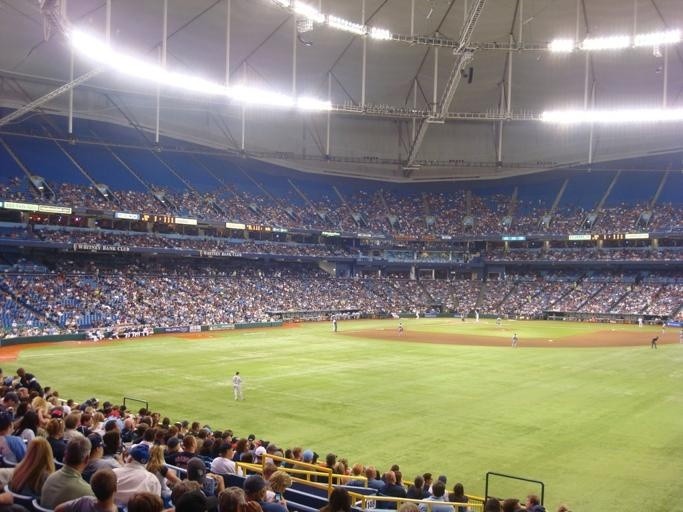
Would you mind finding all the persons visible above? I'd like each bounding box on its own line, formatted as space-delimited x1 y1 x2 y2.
1 367 573 512
1 176 683 346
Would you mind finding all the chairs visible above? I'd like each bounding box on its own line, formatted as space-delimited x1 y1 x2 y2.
0 435 289 512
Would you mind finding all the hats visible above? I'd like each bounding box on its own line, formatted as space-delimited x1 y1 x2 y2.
244 475 269 494
87 433 107 448
304 450 314 460
439 476 447 481
255 446 266 457
0 411 15 421
128 444 150 464
248 434 255 441
135 423 149 431
103 401 113 406
167 437 182 448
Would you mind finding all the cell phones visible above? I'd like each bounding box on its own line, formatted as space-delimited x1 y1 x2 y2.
275 492 281 502
